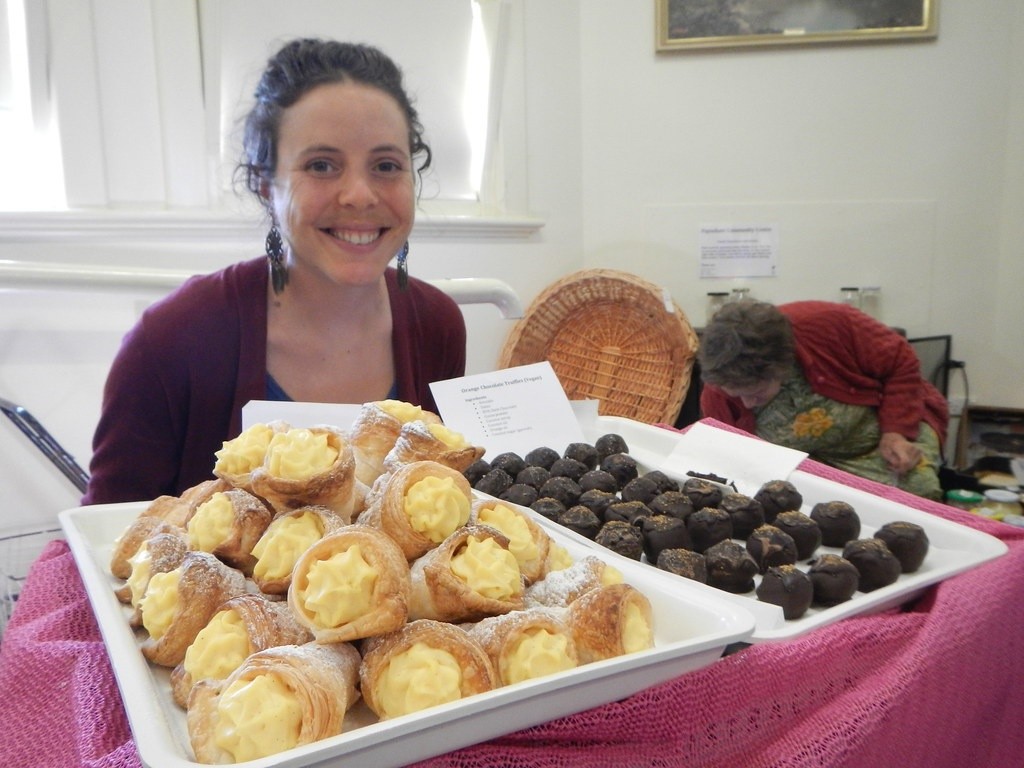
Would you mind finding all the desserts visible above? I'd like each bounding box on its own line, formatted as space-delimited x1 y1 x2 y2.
111 397 654 765
463 432 928 619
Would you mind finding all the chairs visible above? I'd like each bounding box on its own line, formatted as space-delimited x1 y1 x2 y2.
905 331 970 472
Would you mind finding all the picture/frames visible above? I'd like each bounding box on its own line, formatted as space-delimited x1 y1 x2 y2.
652 0 940 51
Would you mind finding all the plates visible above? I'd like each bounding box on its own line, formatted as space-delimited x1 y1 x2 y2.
493 415 1006 643
55 488 753 768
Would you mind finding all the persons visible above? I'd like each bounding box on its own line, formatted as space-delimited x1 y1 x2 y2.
80 38 465 505
700 297 948 501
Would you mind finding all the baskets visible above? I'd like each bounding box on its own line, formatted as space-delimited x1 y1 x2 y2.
502 268 700 427
